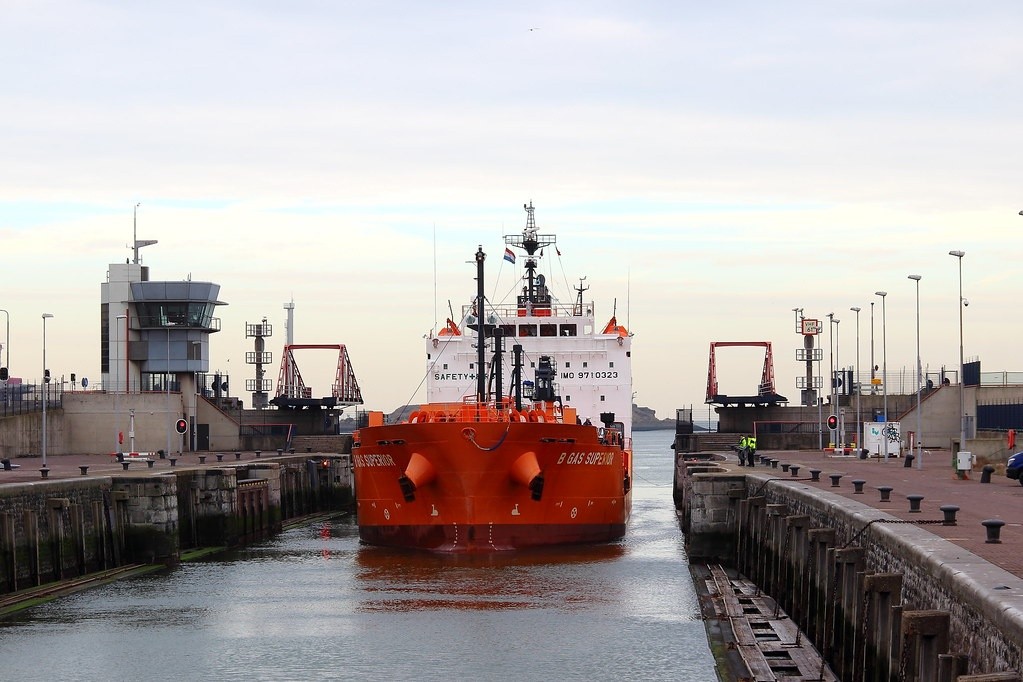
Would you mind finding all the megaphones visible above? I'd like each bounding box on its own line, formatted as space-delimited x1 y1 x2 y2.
586 309 591 314
536 281 540 285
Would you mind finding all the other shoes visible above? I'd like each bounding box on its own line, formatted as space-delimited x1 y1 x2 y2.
746 464 753 467
739 464 744 466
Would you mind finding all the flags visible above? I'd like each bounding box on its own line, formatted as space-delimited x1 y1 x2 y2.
504 247 515 264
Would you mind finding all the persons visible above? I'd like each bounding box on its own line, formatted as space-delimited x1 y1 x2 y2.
745 434 756 467
576 415 592 425
737 434 746 466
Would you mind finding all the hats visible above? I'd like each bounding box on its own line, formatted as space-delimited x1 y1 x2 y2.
739 434 743 436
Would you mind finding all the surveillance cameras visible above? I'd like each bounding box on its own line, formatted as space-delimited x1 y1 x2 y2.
963 301 969 306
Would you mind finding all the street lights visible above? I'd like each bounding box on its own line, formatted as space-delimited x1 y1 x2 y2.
949 250 969 479
827 312 840 451
850 306 861 459
876 292 888 464
162 322 176 460
116 315 127 465
908 275 922 470
38 313 54 468
813 326 822 450
0 310 10 407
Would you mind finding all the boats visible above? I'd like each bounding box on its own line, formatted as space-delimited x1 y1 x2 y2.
351 201 633 553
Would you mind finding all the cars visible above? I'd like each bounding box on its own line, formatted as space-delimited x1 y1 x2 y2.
1005 451 1023 485
